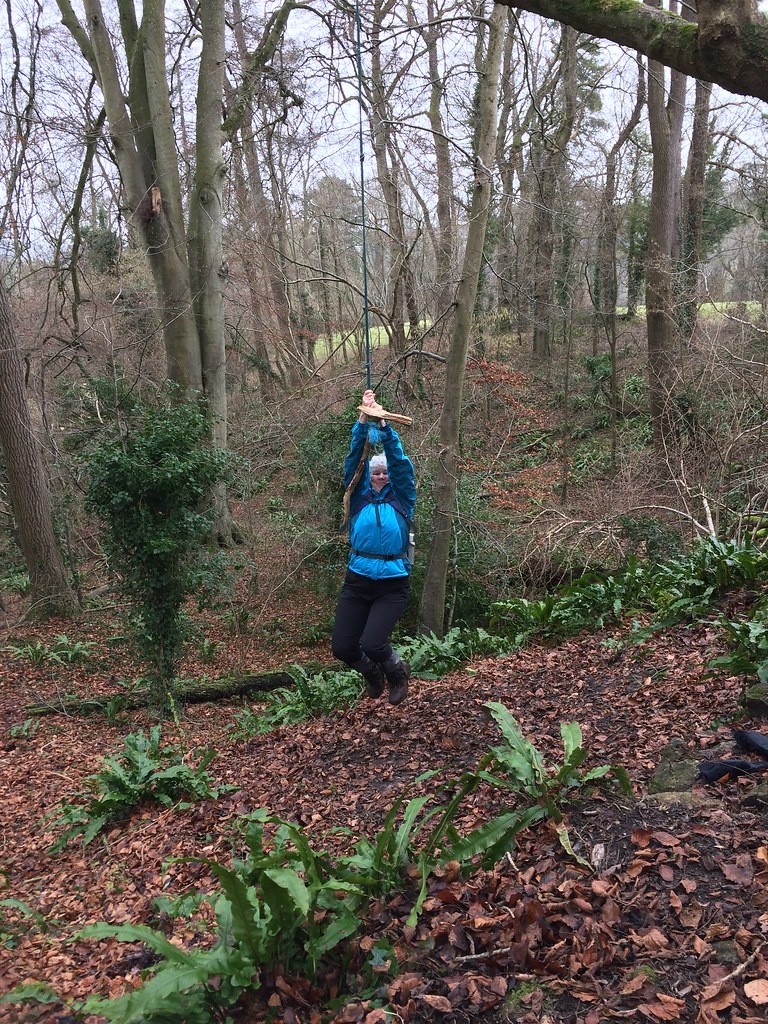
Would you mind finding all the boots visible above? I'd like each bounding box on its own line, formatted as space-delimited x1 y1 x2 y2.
377 648 411 705
351 653 385 698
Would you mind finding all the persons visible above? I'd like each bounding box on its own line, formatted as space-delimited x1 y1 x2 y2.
331 389 417 705
